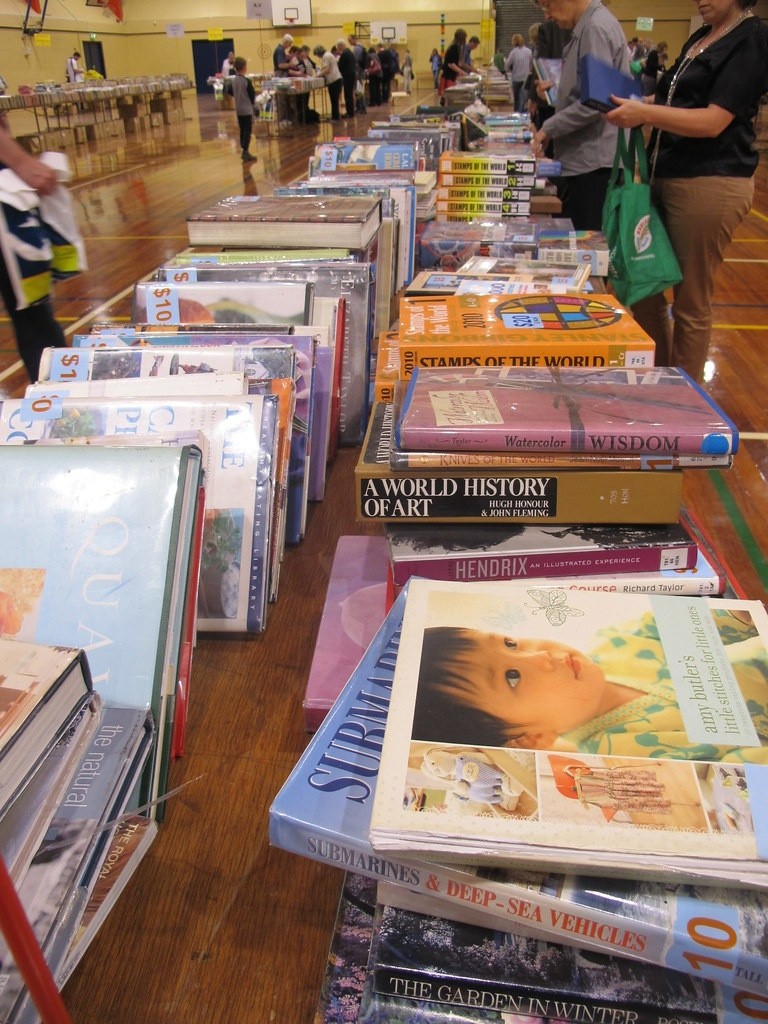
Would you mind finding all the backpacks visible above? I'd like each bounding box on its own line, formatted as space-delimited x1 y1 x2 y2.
356 44 371 69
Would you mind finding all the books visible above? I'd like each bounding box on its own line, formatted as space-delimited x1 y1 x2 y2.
0 106 768 1024
0 74 191 108
445 65 512 104
532 58 563 107
272 77 325 94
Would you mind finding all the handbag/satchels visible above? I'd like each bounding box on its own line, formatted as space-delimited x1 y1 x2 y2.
400 69 414 80
600 124 684 309
368 59 381 73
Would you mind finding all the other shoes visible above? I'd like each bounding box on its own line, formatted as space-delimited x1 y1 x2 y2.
355 110 366 115
342 114 354 119
434 88 439 91
280 120 293 128
329 119 342 123
241 151 257 162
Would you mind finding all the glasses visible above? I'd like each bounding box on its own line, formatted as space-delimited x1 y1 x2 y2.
540 4 550 13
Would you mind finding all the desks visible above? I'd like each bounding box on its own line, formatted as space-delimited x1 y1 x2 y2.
274 86 329 137
0 87 196 144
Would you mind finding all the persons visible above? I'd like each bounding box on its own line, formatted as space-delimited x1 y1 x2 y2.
66 52 85 110
602 1 768 384
488 49 508 79
429 48 442 91
532 0 634 231
528 20 574 159
505 23 547 113
627 37 669 96
0 116 68 386
273 33 412 127
222 52 236 76
226 56 257 161
411 609 768 765
439 29 481 96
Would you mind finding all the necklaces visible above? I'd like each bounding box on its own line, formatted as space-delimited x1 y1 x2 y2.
650 6 754 185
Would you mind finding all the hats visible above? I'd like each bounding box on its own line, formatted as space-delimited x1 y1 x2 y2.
471 36 480 43
